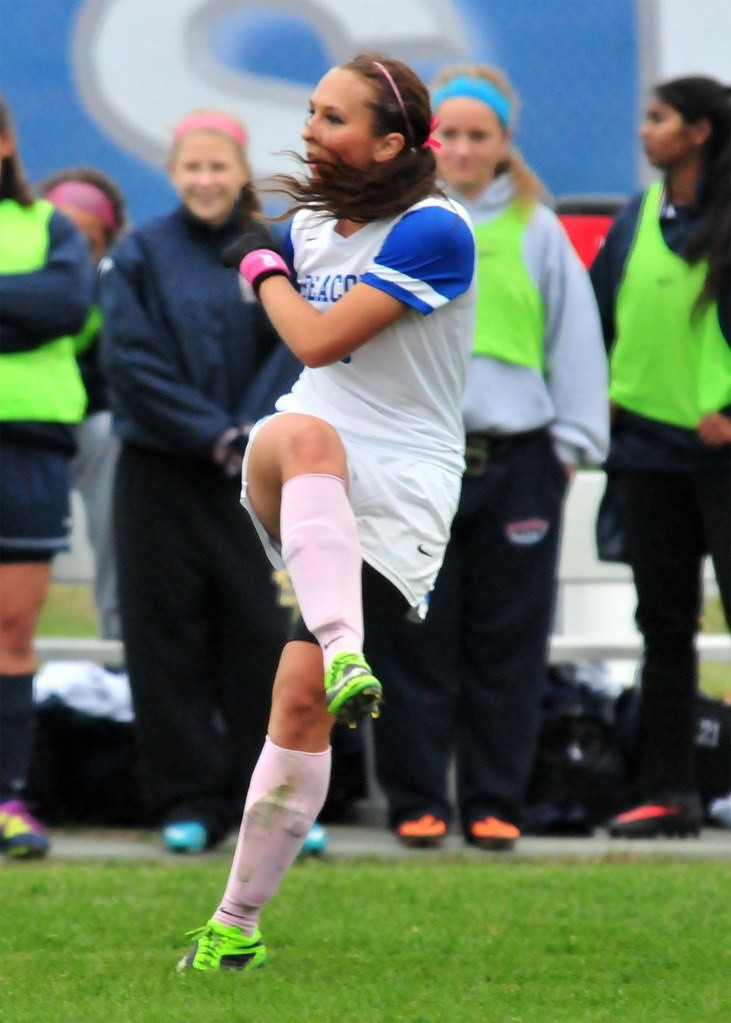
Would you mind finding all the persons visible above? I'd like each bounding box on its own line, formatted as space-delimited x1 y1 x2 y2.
0 95 100 862
368 61 612 864
168 51 477 987
23 168 133 676
88 110 331 858
581 74 731 843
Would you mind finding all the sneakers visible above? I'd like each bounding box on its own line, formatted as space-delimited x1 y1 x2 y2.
173 919 267 984
322 652 384 731
0 799 49 860
609 800 704 836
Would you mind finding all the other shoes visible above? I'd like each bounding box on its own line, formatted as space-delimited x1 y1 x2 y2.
298 820 327 852
472 815 519 852
398 815 446 847
163 819 206 856
